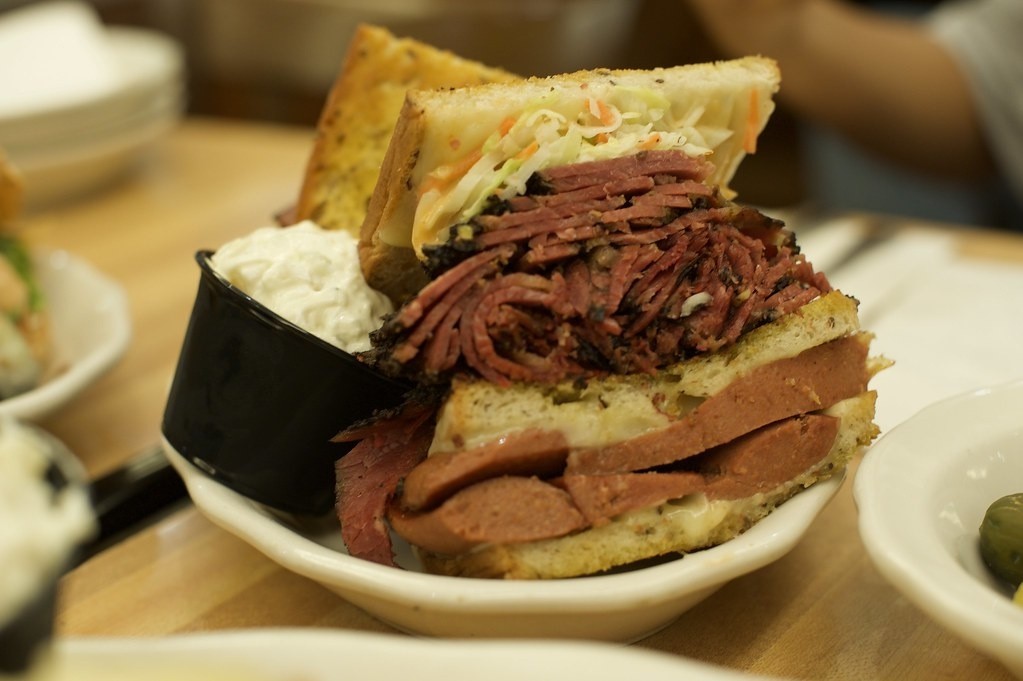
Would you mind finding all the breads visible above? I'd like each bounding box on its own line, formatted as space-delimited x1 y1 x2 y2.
287 27 887 579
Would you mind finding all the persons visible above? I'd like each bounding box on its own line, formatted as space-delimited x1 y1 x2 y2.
694 0 1022 194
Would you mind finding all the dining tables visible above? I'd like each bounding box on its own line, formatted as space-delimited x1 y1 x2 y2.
5 117 1023 677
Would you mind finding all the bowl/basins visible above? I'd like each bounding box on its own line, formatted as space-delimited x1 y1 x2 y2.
159 247 401 521
855 374 1023 681
157 431 849 646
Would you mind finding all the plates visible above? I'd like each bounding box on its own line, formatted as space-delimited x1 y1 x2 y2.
0 239 133 425
0 22 184 206
0 633 777 681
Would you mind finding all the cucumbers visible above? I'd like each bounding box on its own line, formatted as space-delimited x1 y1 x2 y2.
979 492 1023 584
